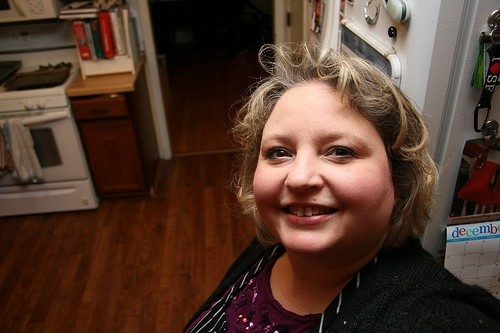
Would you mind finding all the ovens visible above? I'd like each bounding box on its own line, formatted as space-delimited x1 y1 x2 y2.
0 107 100 218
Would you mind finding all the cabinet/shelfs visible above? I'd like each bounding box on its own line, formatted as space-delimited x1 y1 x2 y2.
0 89 100 218
68 55 162 201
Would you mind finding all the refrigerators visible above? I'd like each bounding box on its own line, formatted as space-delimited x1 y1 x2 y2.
302 0 500 267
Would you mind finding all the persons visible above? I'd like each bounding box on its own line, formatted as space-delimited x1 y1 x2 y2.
172 39 500 333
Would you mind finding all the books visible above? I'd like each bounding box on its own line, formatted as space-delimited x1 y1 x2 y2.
73 4 129 62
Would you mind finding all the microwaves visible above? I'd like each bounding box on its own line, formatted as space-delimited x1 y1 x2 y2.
0 0 65 25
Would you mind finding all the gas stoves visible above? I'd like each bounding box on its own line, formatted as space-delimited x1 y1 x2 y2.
0 42 80 111
